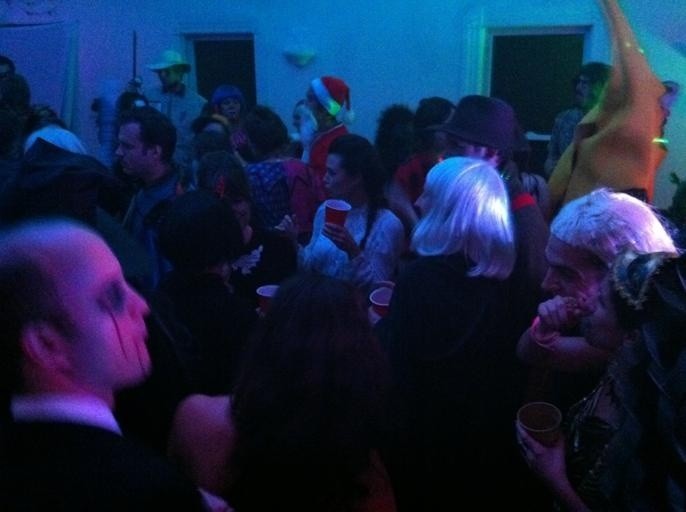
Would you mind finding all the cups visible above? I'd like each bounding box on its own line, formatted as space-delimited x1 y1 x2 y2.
256 285 279 311
323 199 352 227
369 286 394 316
516 401 561 447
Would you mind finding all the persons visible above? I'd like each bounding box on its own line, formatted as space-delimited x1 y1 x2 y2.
0 0 686 511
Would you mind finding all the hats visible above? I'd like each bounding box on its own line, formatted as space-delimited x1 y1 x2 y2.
427 94 530 155
144 51 191 75
311 77 355 125
